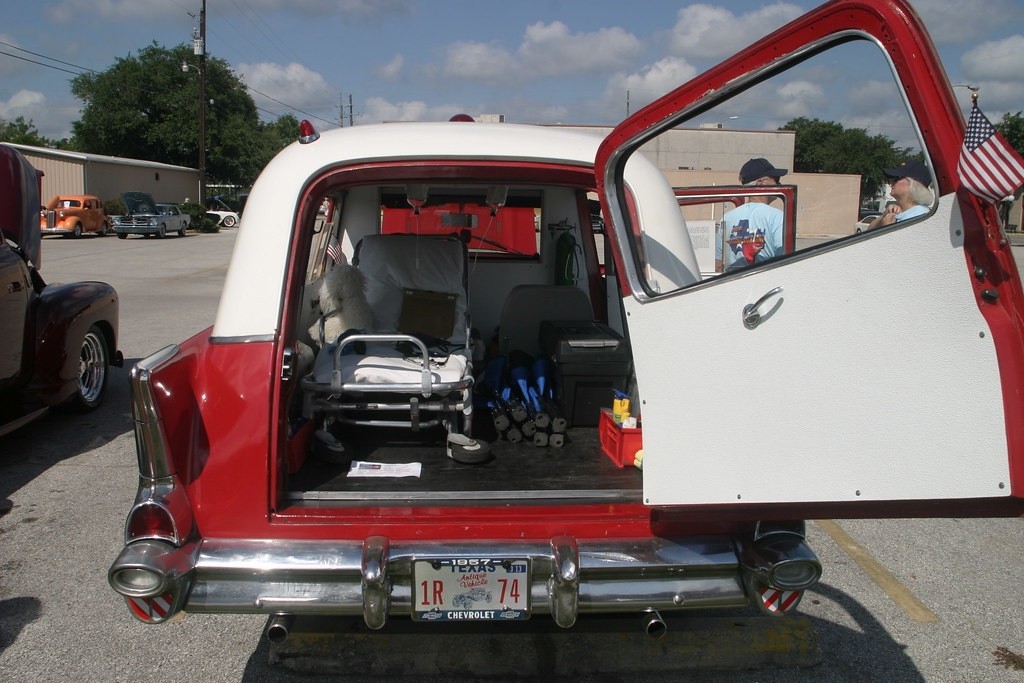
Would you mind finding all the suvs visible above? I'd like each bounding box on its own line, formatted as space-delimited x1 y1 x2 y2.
41 194 109 239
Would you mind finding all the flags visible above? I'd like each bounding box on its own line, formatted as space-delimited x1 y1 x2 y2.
957 103 1024 206
326 232 342 265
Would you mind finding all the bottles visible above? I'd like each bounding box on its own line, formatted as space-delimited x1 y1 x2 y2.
618 412 630 428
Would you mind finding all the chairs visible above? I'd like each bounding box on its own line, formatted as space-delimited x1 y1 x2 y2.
499 284 594 357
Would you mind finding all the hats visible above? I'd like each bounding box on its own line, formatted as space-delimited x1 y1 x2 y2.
883 160 932 188
739 158 788 184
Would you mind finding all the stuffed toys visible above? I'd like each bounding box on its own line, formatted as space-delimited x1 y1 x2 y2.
307 265 376 350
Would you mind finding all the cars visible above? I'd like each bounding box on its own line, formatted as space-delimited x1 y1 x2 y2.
858 211 882 232
0 144 125 458
106 1 1024 646
862 195 898 211
207 211 239 227
112 201 192 240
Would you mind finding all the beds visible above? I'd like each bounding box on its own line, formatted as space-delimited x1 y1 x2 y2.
301 231 489 463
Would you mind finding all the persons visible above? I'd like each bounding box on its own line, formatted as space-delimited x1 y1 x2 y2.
322 197 330 216
714 158 788 277
865 158 934 231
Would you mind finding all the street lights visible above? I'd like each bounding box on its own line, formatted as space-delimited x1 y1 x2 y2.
950 83 981 92
181 61 205 234
722 115 740 122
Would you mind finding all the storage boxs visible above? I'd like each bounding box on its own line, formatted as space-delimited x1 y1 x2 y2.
599 409 642 470
541 319 631 428
